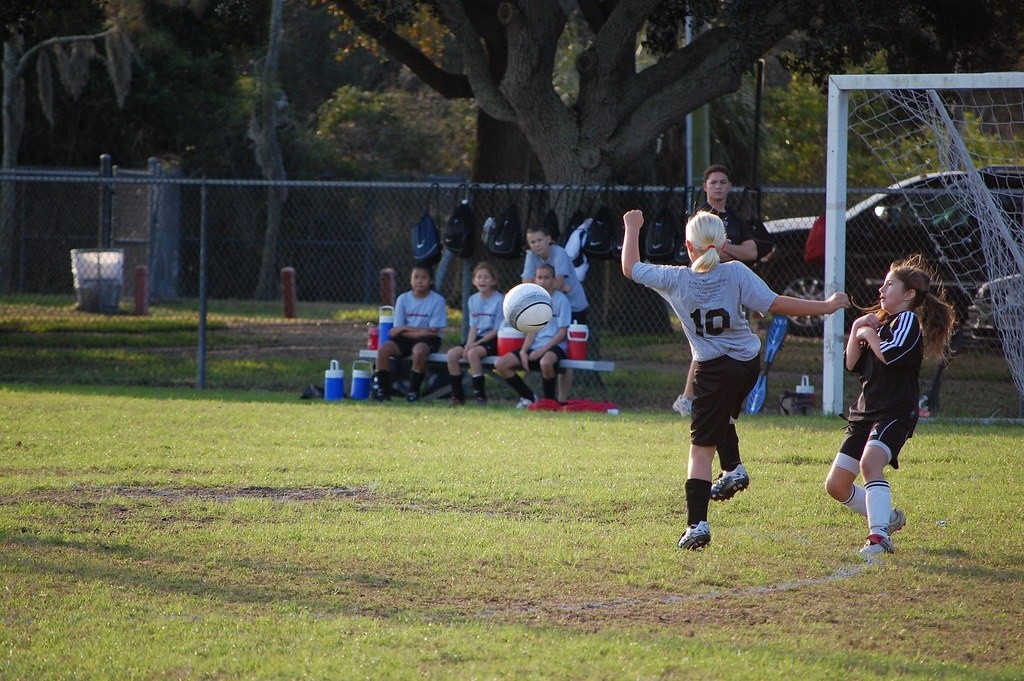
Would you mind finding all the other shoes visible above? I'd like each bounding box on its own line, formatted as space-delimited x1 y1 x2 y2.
516 396 538 408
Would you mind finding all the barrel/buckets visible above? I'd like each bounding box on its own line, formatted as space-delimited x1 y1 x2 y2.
368 321 378 350
351 361 371 399
498 320 524 356
323 360 344 401
379 305 394 348
795 375 816 415
566 320 589 360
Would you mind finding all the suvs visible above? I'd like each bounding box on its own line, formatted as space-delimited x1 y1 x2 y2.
755 164 1024 343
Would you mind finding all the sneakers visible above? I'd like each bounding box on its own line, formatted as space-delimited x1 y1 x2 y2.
678 520 711 550
711 463 749 500
887 509 906 535
672 394 691 416
860 536 893 554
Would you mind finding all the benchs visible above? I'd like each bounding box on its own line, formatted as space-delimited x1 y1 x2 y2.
359 349 615 403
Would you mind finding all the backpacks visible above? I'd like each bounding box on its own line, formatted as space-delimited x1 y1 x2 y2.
408 175 777 267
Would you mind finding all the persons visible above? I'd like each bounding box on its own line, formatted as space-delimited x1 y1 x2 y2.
673 165 758 415
495 263 571 409
448 263 504 407
825 265 955 554
522 226 589 405
621 210 851 550
375 264 447 402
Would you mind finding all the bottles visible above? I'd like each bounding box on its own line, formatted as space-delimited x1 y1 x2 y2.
373 369 385 400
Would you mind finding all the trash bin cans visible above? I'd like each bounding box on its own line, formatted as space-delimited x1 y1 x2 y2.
71 247 125 314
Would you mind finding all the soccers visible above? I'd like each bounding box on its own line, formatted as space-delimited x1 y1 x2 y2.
504 282 551 333
